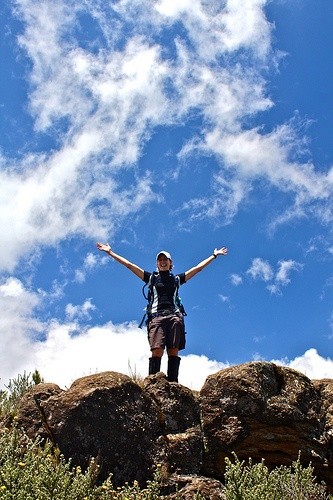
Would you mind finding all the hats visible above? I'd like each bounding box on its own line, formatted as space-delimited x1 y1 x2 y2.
156 251 170 260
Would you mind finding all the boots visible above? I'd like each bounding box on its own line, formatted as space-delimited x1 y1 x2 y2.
149 358 160 374
168 356 181 382
